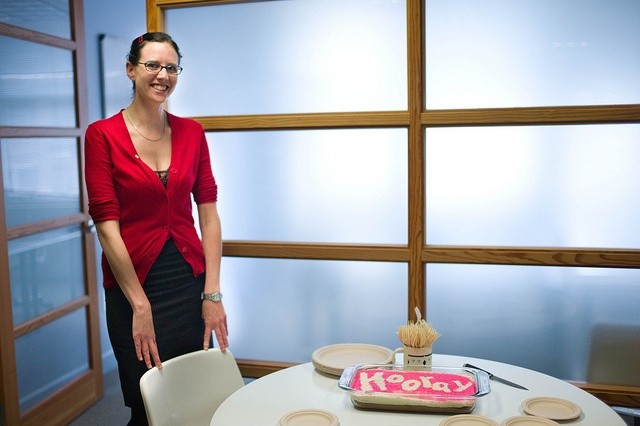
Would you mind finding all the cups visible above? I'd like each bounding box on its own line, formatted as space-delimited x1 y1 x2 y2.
392 344 433 370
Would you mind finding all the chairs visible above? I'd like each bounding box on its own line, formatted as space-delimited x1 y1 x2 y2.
140 348 244 426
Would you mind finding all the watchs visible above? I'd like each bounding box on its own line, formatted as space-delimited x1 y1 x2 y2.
200 293 223 303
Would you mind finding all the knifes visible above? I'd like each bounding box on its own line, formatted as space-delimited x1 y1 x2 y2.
462 363 529 390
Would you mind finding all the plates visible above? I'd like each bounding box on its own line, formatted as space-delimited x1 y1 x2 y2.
279 408 339 426
521 396 582 421
501 415 560 425
438 414 500 426
312 342 395 376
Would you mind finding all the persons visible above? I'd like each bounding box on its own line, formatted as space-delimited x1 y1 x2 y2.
84 31 229 426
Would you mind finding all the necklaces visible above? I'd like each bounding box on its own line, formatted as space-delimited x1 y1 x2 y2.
124 106 167 142
